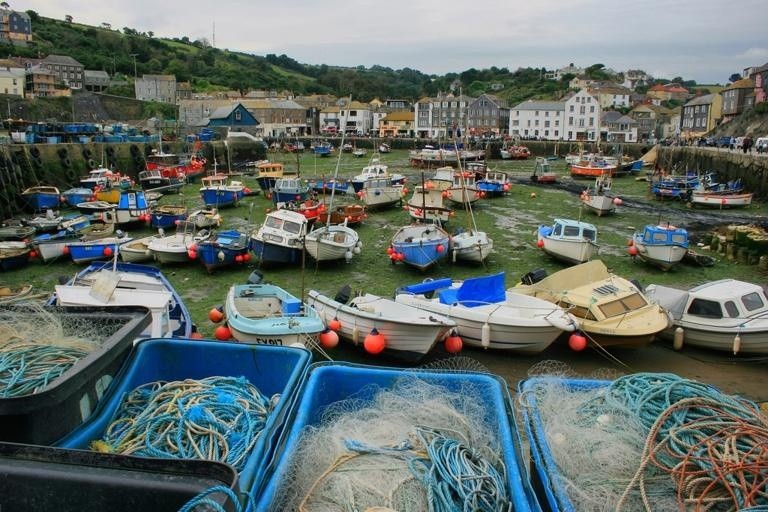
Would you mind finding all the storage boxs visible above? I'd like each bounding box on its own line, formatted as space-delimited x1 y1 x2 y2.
11 122 159 143
187 128 220 143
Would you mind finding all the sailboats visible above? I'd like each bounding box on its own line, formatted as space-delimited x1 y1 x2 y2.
448 138 494 263
303 92 360 270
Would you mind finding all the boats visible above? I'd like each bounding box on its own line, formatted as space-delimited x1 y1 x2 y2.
505 257 669 352
117 227 167 264
627 222 689 272
390 171 452 274
146 220 210 267
631 275 768 367
394 270 580 358
580 173 623 217
530 156 557 184
305 283 457 368
536 215 602 269
209 234 327 353
564 127 636 180
0 136 531 271
248 207 309 271
648 147 754 209
65 229 134 265
195 225 253 275
3 118 218 143
41 244 193 347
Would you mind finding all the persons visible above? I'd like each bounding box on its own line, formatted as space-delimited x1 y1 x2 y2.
661 133 764 153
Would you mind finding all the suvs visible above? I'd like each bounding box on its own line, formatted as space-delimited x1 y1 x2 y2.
660 135 768 152
